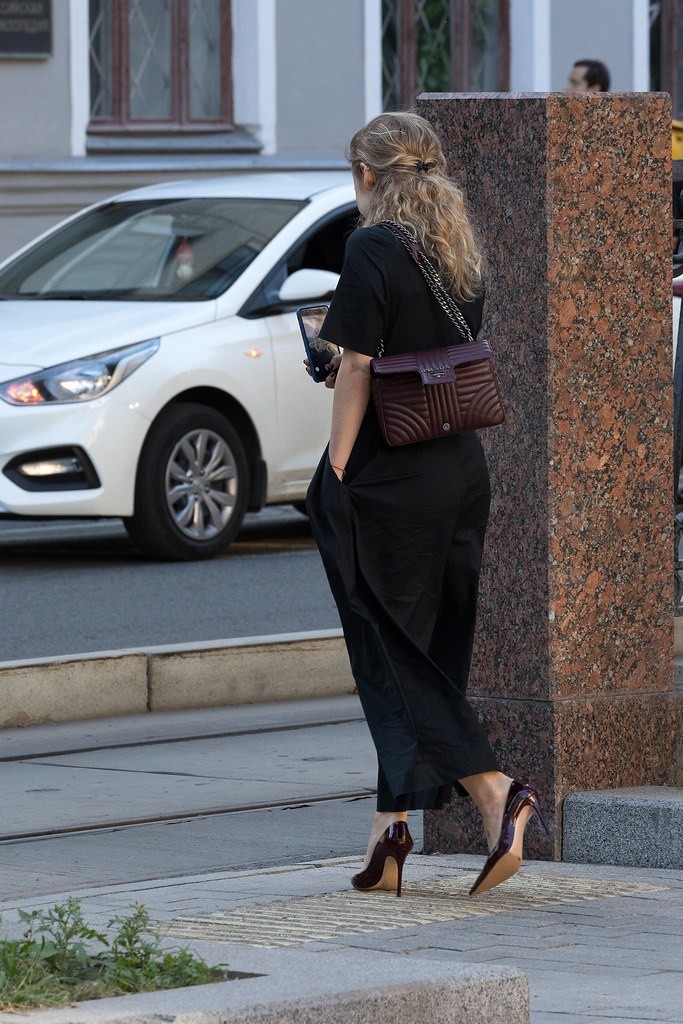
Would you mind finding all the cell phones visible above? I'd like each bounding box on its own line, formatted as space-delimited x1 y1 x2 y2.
296 305 341 383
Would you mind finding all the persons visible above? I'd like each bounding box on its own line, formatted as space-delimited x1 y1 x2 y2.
307 332 338 372
303 111 551 898
568 59 610 93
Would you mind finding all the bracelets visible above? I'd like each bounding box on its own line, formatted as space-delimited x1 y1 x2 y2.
331 464 345 473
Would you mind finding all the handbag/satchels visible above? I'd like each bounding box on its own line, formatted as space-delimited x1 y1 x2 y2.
368 219 506 446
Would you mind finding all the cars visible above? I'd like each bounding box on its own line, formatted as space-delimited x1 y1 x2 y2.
0 171 366 562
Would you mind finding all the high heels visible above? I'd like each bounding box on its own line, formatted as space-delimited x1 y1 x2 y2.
469 780 550 896
350 821 414 897
